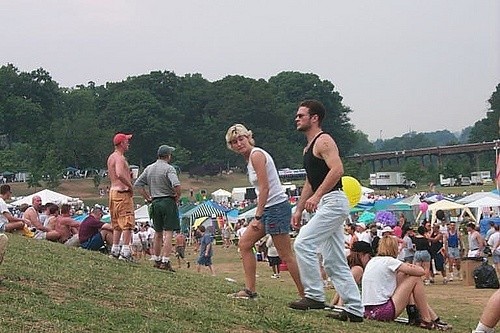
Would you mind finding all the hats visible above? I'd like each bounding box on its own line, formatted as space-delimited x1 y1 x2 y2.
351 241 372 253
356 222 365 229
158 145 176 156
382 227 394 233
114 134 132 146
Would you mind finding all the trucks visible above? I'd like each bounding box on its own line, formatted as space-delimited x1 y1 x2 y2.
469 171 491 185
439 173 459 186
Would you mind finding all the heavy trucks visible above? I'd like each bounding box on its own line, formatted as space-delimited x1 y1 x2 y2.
369 170 417 190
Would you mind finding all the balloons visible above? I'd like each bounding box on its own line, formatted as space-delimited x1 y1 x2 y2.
419 202 428 213
393 226 402 237
341 176 362 207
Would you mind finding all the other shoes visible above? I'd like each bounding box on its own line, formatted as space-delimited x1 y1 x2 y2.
458 274 463 281
154 260 162 267
449 272 453 280
326 310 363 322
186 261 190 268
160 261 176 272
286 297 323 310
119 254 137 263
109 251 120 258
436 319 447 325
443 277 448 284
420 320 448 331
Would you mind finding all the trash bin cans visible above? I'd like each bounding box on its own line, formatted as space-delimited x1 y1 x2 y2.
460 257 483 286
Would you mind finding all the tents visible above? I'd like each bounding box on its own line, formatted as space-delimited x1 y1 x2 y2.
10 189 500 244
0 165 139 182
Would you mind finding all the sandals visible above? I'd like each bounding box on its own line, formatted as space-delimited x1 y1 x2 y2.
227 289 257 300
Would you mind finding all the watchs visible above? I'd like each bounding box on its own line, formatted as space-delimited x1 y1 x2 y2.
255 215 263 220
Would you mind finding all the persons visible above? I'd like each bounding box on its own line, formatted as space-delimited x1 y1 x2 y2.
223 124 305 300
23 195 61 242
0 199 500 333
0 185 31 231
107 133 138 264
0 175 14 184
134 145 182 272
368 182 474 199
290 100 363 322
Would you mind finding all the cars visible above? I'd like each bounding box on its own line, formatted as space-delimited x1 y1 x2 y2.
481 176 493 185
461 177 471 186
